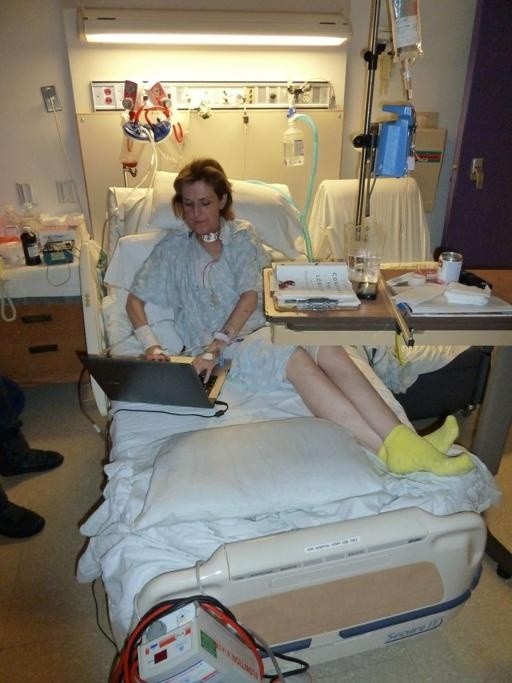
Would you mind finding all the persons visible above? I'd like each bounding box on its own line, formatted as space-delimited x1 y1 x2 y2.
1 371 64 537
125 158 471 473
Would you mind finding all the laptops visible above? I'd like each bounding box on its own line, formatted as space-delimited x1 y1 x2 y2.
75 349 232 408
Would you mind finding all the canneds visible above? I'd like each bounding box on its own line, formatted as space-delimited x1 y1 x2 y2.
435 251 464 286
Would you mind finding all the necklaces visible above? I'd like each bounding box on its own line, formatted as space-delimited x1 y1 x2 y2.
199 230 220 244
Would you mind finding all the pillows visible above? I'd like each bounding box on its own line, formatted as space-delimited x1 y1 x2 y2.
146 171 302 262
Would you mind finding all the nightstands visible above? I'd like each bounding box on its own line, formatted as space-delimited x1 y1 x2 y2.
0 241 109 417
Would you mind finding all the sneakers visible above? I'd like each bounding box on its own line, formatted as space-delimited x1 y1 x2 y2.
0 430 65 474
0 495 45 539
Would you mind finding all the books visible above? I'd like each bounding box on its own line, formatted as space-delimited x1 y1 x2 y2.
268 261 359 311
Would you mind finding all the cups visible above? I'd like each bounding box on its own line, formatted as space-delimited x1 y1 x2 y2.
408 274 426 286
346 223 386 300
119 119 184 168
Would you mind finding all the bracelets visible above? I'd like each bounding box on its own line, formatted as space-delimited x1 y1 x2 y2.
210 331 231 343
134 326 161 352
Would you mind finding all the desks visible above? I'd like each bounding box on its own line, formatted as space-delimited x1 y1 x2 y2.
262 267 512 476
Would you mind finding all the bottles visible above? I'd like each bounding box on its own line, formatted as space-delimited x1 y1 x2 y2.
0 202 42 266
283 121 305 167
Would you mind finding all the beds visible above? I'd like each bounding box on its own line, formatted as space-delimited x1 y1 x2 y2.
80 171 504 681
